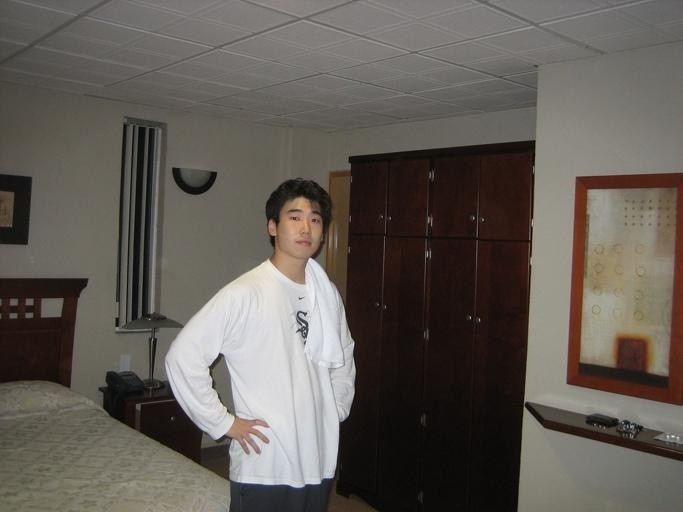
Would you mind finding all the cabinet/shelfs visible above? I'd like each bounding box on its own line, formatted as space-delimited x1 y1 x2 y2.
424 145 534 512
337 149 431 512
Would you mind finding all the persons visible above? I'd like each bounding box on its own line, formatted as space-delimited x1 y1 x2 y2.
163 175 359 512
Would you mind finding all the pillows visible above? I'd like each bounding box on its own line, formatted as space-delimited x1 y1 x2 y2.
1 380 109 422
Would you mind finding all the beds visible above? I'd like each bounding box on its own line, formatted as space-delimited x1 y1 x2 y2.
1 280 232 512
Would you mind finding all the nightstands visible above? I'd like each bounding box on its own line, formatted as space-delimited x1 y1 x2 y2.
98 381 202 464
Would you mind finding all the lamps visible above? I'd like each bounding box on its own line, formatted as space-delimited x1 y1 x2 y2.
120 311 184 389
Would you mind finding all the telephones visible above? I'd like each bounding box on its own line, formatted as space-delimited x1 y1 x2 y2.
106 370 144 396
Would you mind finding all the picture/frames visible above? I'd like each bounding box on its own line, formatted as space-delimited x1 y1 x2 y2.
0 173 32 244
566 173 683 410
